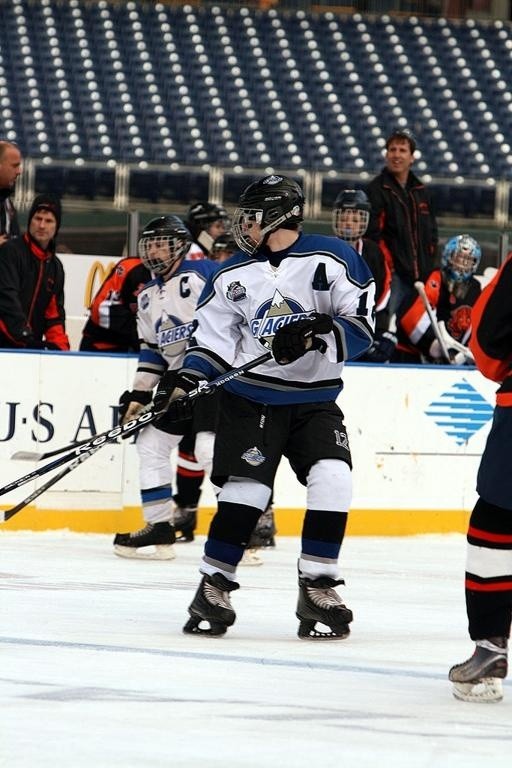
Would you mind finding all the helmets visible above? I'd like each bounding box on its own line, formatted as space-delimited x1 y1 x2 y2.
138 215 192 271
442 234 483 281
331 187 371 241
190 202 239 252
230 175 305 256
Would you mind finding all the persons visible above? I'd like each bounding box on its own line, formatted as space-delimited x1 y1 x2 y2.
173 436 204 530
113 213 278 549
448 252 512 686
0 141 71 352
150 173 380 628
80 203 238 355
330 130 482 366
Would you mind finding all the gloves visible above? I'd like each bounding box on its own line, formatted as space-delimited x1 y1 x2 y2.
151 376 200 425
270 312 335 366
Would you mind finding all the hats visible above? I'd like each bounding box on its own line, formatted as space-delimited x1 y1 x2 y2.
28 195 65 230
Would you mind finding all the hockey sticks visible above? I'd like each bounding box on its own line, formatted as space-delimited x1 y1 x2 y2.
11 403 157 461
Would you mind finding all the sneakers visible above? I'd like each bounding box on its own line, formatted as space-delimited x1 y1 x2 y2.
243 508 277 549
448 635 507 685
168 508 199 533
295 575 354 625
113 521 179 547
188 572 237 626
